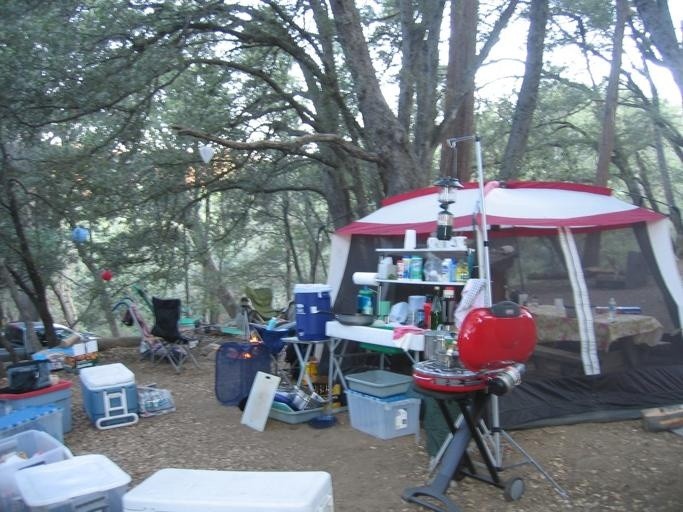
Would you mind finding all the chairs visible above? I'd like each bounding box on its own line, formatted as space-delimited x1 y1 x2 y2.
241 287 286 341
130 284 205 374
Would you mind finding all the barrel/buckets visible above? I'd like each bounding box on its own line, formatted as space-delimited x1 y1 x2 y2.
293 284 334 342
388 301 409 324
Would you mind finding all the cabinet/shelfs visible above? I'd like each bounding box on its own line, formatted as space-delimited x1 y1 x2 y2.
374 246 470 286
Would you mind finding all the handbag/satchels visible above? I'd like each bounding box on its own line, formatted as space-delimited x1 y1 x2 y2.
0 358 52 395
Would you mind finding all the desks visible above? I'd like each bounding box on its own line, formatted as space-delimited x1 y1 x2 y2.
325 319 456 415
526 304 661 354
282 335 352 415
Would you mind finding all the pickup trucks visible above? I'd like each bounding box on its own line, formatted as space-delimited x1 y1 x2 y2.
0 322 101 372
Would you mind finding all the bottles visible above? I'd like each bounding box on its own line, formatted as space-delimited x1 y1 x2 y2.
417 285 456 331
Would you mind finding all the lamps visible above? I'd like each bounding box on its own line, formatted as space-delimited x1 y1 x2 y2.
434 148 466 240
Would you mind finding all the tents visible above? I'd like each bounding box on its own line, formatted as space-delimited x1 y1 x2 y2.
319 180 682 432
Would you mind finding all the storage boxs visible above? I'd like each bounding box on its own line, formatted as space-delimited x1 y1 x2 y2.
5 359 53 393
268 391 325 425
78 362 140 430
0 375 74 435
121 467 334 512
1 430 75 512
343 388 422 440
14 453 133 512
137 383 176 418
0 403 66 445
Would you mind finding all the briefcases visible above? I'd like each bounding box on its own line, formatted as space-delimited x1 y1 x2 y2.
411 300 539 394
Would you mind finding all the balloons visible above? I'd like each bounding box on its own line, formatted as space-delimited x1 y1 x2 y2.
102 269 112 282
72 226 88 245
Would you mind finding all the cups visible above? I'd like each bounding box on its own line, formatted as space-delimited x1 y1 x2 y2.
426 236 467 248
